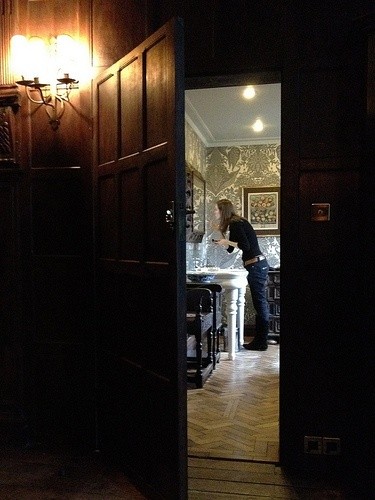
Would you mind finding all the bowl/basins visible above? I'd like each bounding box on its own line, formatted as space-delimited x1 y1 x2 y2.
186 274 216 283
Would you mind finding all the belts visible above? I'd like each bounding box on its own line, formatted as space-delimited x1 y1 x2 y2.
244 255 266 268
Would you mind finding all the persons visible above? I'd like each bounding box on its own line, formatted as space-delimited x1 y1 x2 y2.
215 199 270 350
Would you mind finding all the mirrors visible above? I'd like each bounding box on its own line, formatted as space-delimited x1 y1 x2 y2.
192 165 206 236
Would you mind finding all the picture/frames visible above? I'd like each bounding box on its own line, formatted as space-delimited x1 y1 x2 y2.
243 188 280 235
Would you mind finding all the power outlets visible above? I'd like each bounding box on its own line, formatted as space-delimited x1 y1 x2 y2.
322 437 341 455
304 436 321 455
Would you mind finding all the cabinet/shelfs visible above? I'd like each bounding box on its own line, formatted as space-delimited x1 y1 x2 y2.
267 271 280 345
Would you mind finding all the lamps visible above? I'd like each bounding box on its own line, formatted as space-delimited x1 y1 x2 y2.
9 34 80 125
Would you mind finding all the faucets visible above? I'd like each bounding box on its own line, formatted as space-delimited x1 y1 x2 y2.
200 257 210 268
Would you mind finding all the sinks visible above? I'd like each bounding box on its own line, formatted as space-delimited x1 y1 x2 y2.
187 265 249 289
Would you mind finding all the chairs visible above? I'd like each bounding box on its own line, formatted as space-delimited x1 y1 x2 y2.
184 282 224 388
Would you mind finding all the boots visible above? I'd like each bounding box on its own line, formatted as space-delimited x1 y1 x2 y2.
242 315 268 351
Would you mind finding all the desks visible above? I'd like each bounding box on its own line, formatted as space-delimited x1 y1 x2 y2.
186 267 248 360
